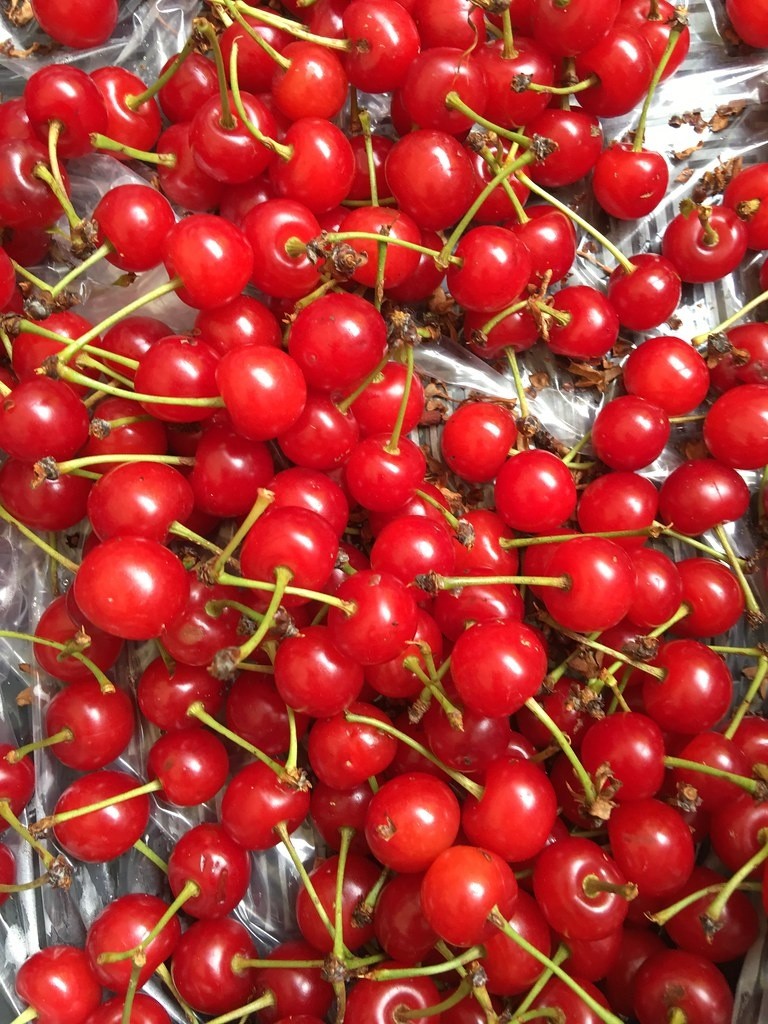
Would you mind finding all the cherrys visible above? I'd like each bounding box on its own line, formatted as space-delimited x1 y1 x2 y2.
0 0 768 1024
